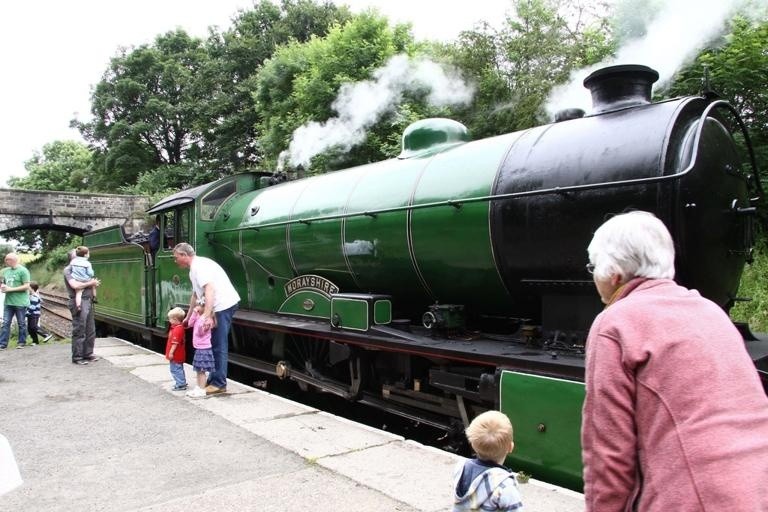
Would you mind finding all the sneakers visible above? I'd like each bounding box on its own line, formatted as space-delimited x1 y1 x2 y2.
76 359 87 365
30 342 36 346
88 356 98 362
16 345 24 349
205 383 226 393
187 386 206 397
92 296 98 303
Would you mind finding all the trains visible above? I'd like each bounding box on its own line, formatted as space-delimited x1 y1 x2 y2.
80 62 767 498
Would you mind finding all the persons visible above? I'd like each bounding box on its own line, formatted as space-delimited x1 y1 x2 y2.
149 215 172 264
65 246 98 313
581 210 768 510
455 411 524 512
0 253 31 349
173 243 241 393
185 296 216 397
166 308 188 391
26 283 52 346
63 249 101 365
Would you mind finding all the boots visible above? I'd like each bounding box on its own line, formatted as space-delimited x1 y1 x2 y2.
43 334 53 342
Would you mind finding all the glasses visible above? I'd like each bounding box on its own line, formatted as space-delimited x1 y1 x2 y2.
586 263 596 273
